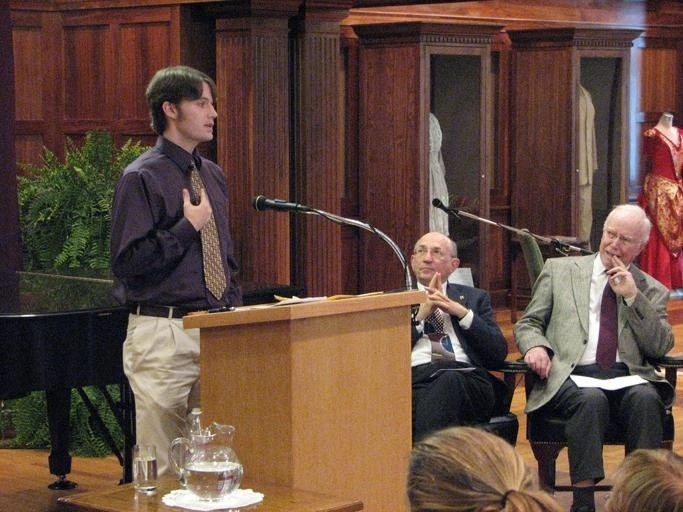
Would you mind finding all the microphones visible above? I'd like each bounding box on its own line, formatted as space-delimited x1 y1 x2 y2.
432 198 463 223
252 195 313 212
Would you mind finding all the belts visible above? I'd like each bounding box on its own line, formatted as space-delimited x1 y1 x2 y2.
127 302 219 319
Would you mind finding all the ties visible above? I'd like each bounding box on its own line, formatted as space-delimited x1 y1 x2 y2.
424 307 456 365
189 158 228 302
595 269 619 373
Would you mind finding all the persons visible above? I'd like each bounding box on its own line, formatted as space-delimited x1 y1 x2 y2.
409 231 509 446
604 448 683 512
643 113 683 291
111 64 243 473
406 424 562 512
512 203 676 512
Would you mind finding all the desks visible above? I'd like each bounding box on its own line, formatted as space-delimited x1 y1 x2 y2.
506 235 589 325
54 471 366 512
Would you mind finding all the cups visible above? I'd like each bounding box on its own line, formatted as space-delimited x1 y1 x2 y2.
131 442 158 493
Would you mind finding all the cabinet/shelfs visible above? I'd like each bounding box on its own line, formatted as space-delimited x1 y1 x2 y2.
506 23 648 311
349 19 503 306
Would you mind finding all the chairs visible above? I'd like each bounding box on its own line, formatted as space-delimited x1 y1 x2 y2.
485 360 526 447
517 349 682 497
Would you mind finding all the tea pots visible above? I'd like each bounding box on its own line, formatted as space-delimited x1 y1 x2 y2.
167 422 245 502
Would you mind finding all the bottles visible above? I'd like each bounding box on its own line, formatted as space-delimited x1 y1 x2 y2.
179 406 206 490
